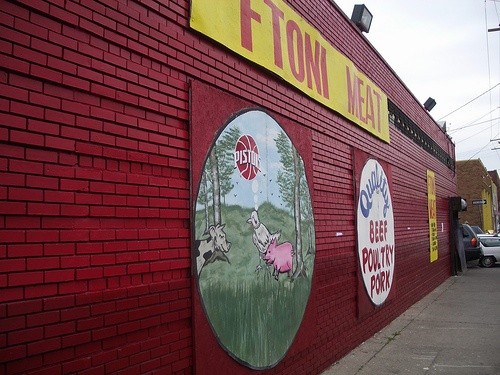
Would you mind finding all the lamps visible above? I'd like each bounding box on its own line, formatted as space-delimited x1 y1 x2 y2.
425 97 436 112
352 3 374 33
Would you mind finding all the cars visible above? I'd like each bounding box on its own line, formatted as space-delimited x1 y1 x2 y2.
461 223 500 268
